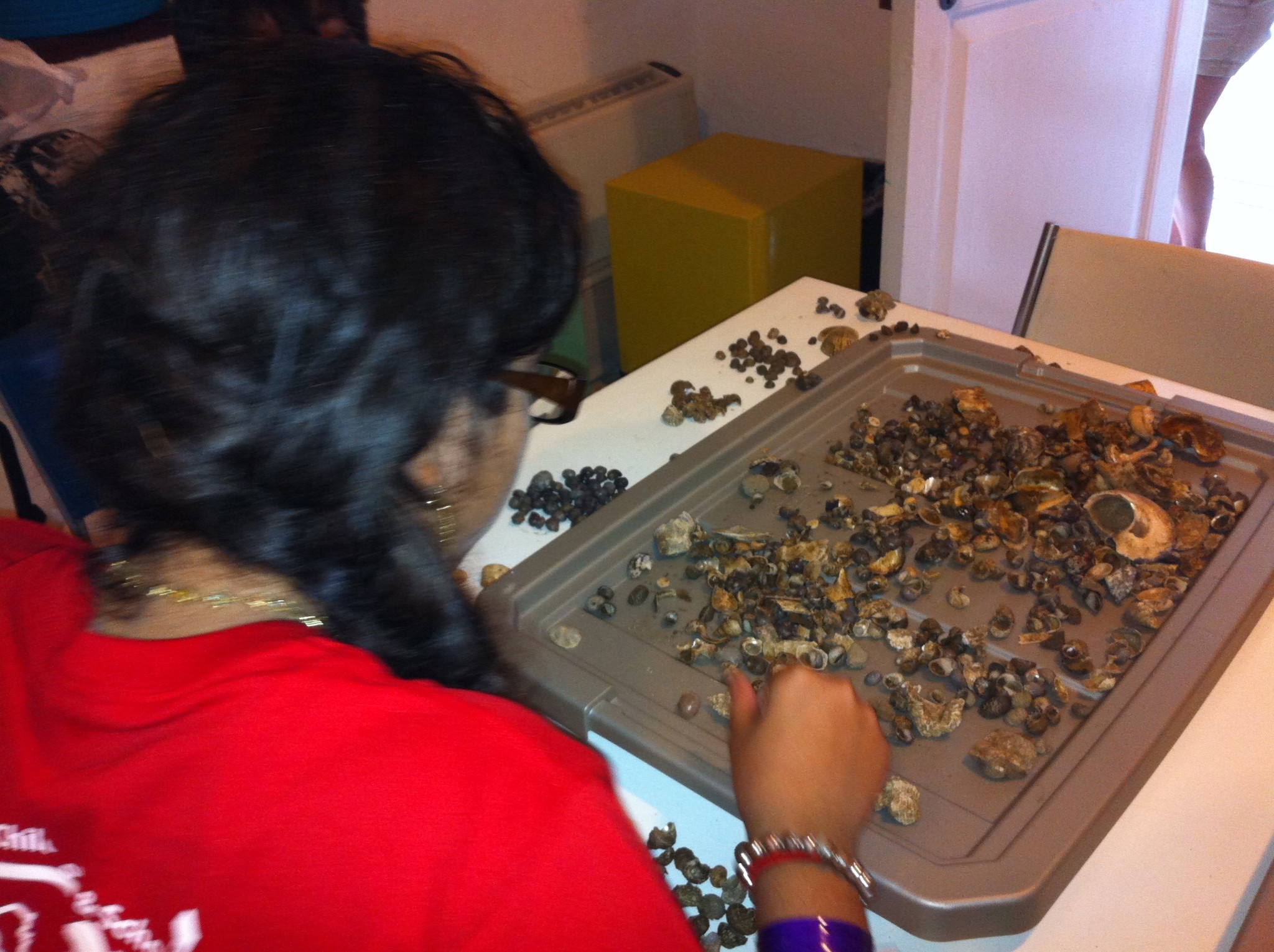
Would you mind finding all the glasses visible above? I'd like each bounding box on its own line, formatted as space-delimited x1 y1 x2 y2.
479 353 589 425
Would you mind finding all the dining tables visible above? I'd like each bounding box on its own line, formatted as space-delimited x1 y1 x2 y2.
453 275 1274 952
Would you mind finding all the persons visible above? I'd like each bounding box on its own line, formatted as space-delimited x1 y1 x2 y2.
1168 0 1274 251
0 36 896 952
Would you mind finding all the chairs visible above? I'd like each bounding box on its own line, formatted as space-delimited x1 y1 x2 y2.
1011 223 1274 410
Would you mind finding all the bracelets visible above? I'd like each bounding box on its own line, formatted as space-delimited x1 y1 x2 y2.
736 833 873 906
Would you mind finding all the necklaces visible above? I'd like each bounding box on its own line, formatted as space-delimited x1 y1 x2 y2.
107 559 330 627
